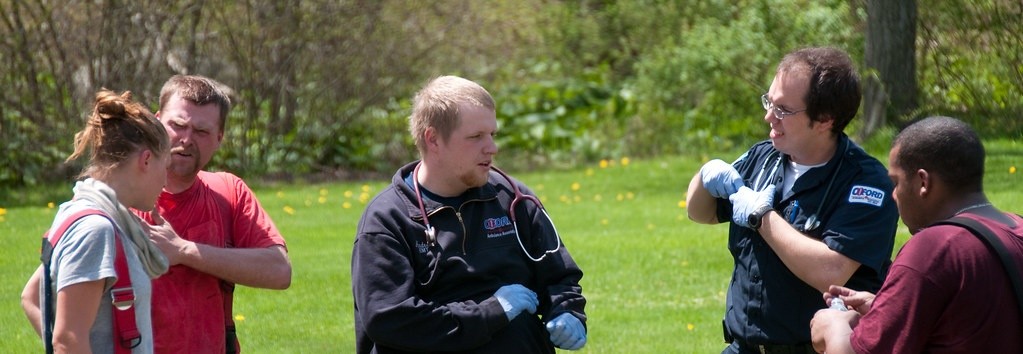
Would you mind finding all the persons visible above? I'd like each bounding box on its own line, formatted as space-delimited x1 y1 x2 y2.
350 75 587 354
811 117 1023 354
124 75 294 354
685 43 899 354
21 85 172 354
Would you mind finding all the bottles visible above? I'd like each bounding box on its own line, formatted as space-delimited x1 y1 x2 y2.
824 298 848 354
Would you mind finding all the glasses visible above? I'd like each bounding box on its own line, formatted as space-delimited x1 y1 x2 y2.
761 93 807 119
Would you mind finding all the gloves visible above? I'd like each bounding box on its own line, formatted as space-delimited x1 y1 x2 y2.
546 313 587 350
700 159 745 199
729 185 776 228
495 283 541 322
